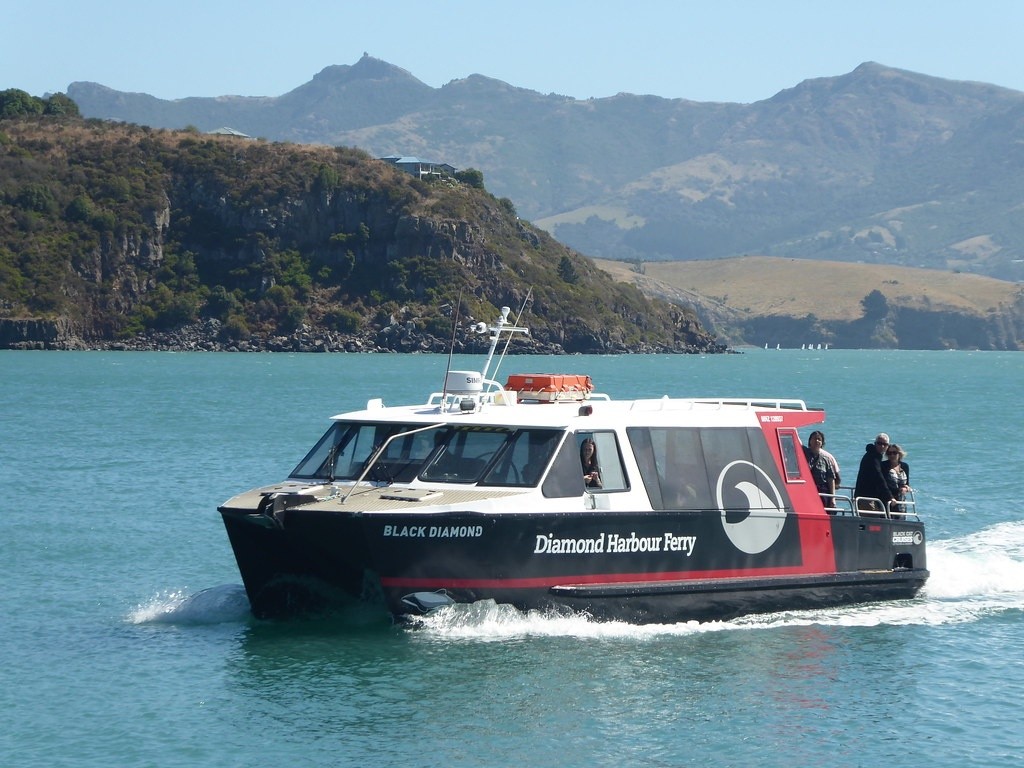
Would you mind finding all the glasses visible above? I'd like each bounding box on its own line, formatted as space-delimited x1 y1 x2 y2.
874 441 889 447
886 451 900 456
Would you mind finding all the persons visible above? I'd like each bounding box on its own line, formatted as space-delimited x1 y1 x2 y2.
579 439 603 488
668 469 696 508
854 433 913 521
802 431 841 515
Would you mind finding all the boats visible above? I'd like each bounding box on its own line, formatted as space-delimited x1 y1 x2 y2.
217 285 929 620
